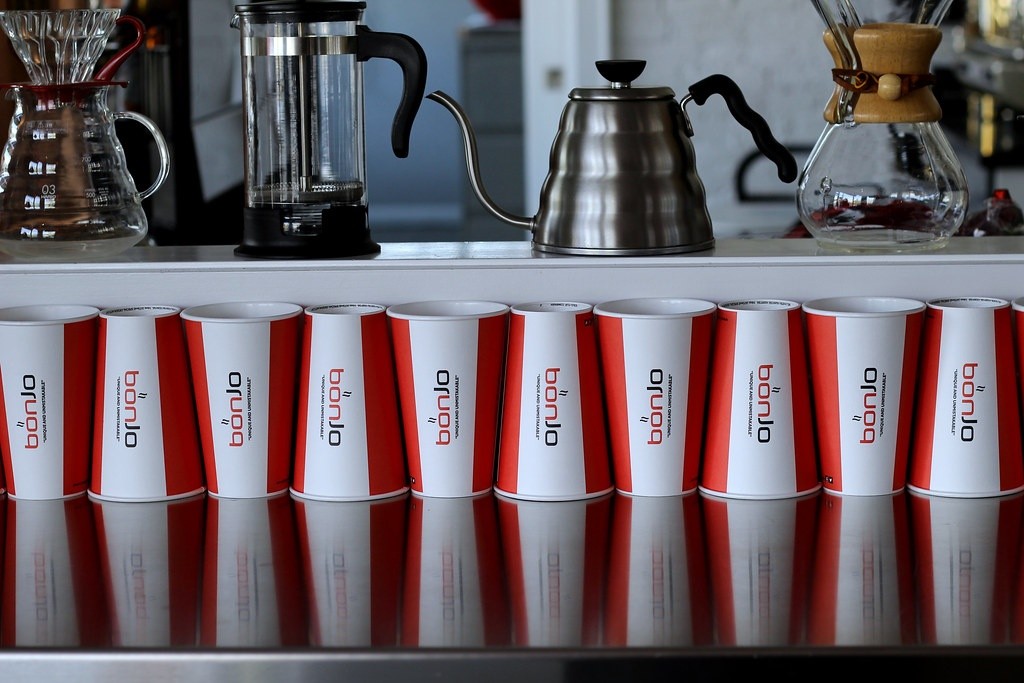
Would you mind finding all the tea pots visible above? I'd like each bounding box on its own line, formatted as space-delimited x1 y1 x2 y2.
423 59 798 259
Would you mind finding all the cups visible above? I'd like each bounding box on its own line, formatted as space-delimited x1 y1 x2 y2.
1 497 1024 649
0 8 145 84
0 300 1023 501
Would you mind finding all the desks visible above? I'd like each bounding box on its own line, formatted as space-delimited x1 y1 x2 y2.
0 485 1024 683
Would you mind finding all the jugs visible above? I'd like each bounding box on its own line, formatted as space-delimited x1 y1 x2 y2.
0 79 171 263
228 1 428 261
